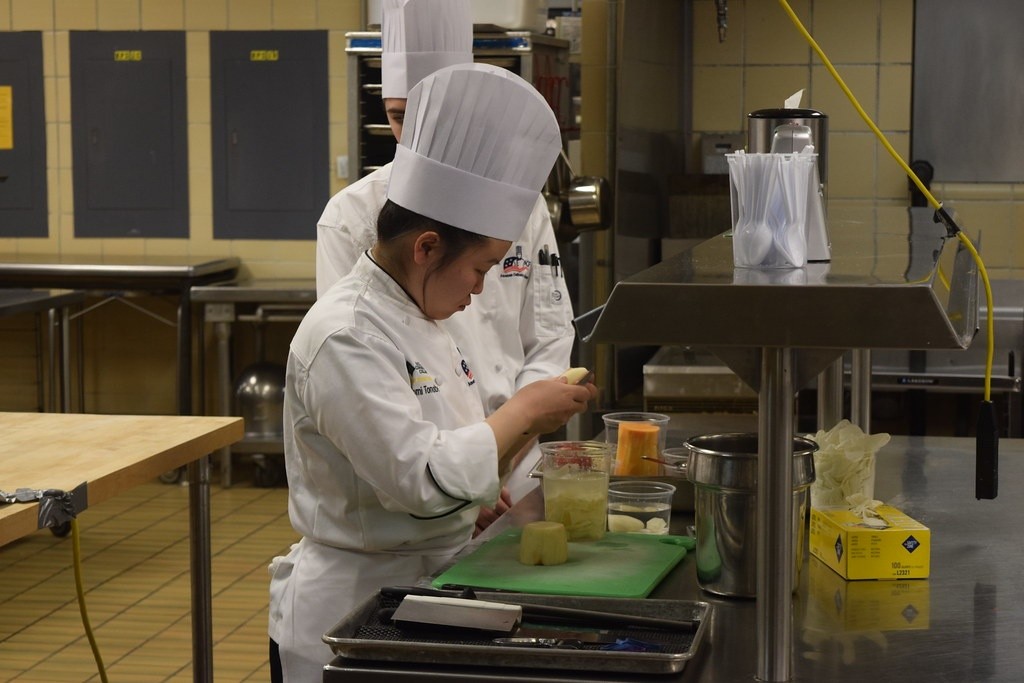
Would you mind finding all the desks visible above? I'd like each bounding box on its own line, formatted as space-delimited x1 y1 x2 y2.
0 287 87 539
0 254 240 484
0 412 246 682
323 426 1024 683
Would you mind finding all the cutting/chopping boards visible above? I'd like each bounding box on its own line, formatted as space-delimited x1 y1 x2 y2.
429 526 697 599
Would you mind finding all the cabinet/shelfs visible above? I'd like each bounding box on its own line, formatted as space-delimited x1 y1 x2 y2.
191 278 317 489
0 29 330 241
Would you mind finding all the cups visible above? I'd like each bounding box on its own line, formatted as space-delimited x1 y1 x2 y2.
539 440 617 542
606 480 678 535
724 153 819 270
601 411 670 477
660 447 694 480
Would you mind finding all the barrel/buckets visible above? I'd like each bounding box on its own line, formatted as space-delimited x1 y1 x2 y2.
681 432 822 598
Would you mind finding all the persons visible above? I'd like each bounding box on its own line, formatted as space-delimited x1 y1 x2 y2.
317 0 575 539
267 64 596 683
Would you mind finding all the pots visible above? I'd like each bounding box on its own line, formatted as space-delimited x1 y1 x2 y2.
542 146 612 244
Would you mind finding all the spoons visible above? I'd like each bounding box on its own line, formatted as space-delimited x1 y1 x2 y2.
728 144 815 267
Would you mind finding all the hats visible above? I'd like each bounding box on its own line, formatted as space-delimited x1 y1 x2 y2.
381 0 474 100
387 62 562 242
